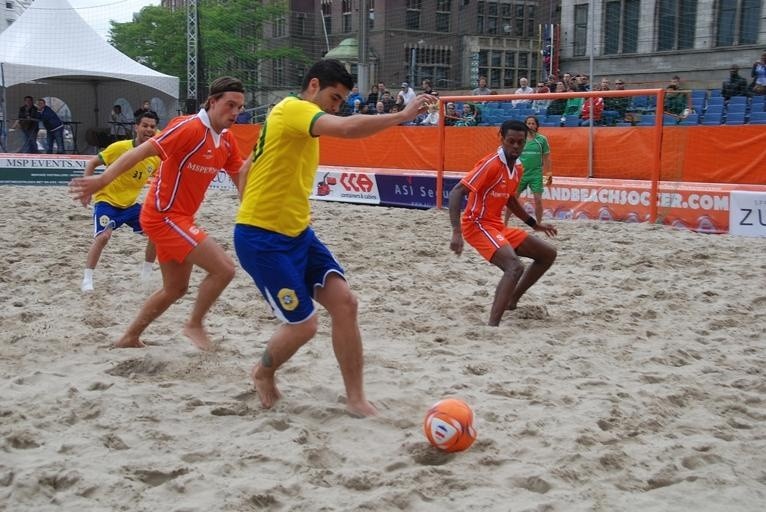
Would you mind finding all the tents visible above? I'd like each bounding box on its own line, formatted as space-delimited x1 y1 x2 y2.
0 1 180 154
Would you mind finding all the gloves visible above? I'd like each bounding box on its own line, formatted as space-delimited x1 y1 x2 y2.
546 172 554 188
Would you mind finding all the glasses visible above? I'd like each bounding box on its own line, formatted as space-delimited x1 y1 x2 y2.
539 86 544 87
616 82 623 86
448 108 454 109
463 107 470 109
430 94 438 97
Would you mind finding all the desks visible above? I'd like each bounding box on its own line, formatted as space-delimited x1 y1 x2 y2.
105 121 135 142
53 120 82 155
7 117 41 153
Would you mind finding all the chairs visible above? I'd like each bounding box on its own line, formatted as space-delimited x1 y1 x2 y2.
441 88 766 124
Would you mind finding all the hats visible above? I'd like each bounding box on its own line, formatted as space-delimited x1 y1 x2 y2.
401 83 408 87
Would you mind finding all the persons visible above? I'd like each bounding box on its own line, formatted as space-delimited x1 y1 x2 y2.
348 99 361 115
511 77 534 109
484 91 502 109
345 86 365 109
413 92 429 123
541 38 555 83
269 103 276 111
364 85 379 113
422 79 432 94
108 104 129 143
395 95 406 112
445 103 460 126
671 75 692 114
232 57 439 419
18 96 40 153
133 100 153 118
420 104 439 126
720 64 748 106
398 82 416 105
358 102 372 115
664 84 687 119
78 112 162 293
504 116 554 227
37 98 65 154
453 103 477 126
377 82 389 101
380 91 395 113
430 91 438 97
448 119 558 327
391 105 399 113
67 75 245 350
602 78 629 127
748 50 766 96
531 68 610 128
472 77 492 104
372 102 385 115
234 105 253 124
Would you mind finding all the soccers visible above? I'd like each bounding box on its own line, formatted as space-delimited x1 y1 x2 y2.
425 399 475 452
